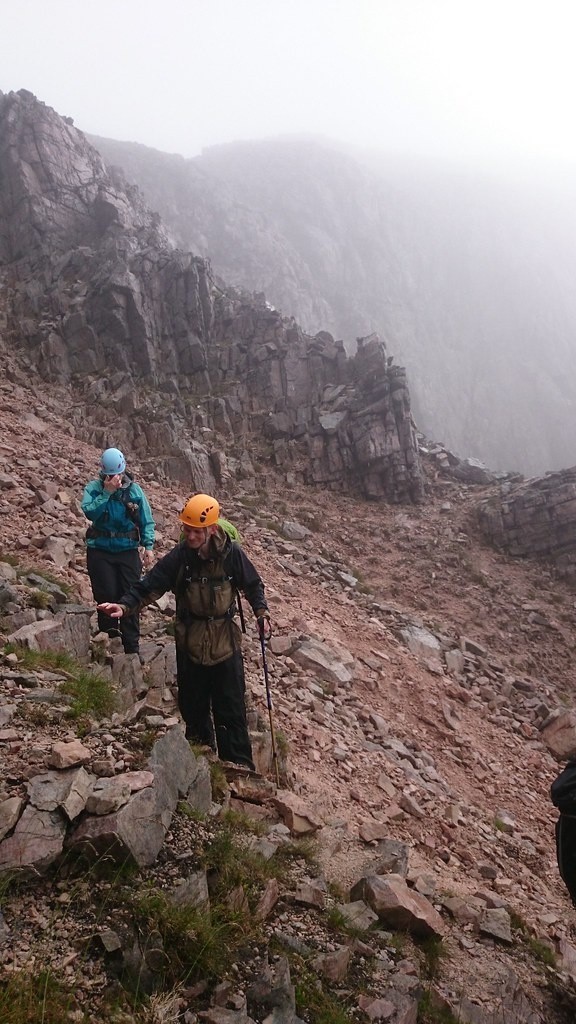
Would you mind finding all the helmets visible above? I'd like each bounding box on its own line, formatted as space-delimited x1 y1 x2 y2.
101 448 125 474
178 493 220 527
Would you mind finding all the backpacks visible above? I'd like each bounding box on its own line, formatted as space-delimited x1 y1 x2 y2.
551 760 576 909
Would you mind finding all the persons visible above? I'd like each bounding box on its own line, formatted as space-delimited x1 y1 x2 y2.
94 494 272 767
80 448 157 665
